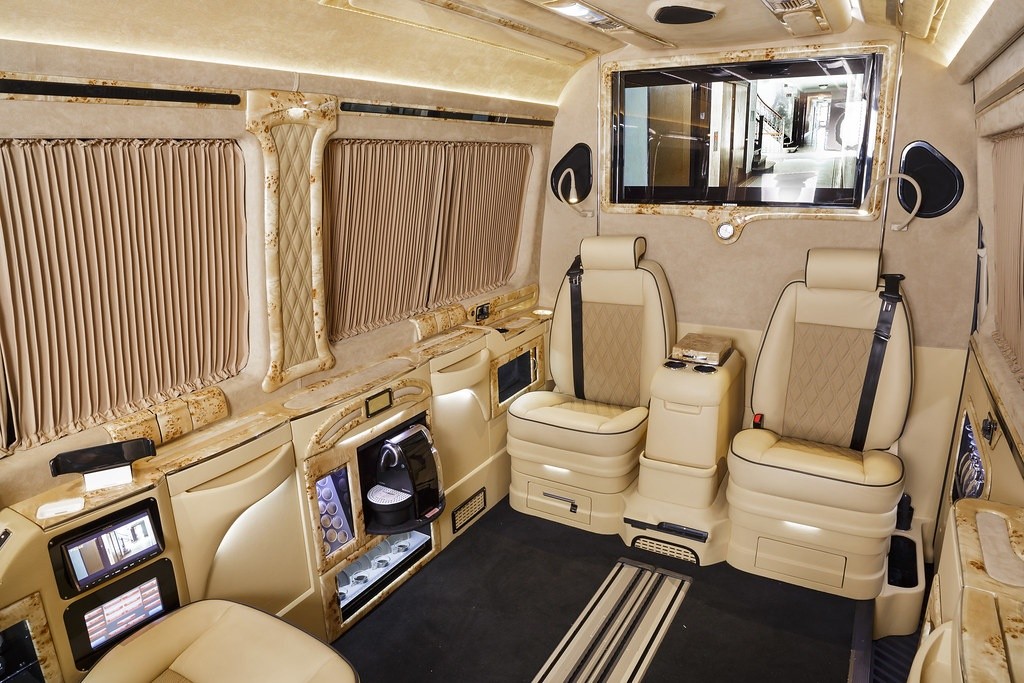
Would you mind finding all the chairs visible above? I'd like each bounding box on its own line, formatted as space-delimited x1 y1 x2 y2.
506 237 678 535
81 599 360 683
726 249 916 600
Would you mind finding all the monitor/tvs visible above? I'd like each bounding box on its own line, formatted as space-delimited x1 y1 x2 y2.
611 53 883 209
497 348 538 404
60 508 167 651
